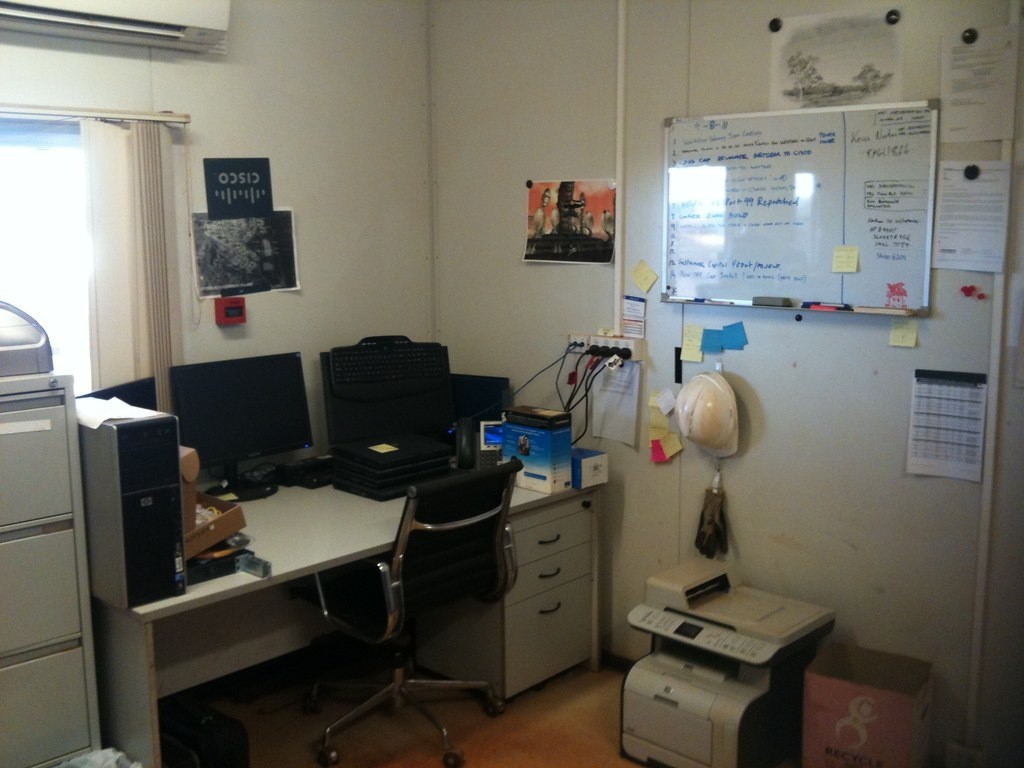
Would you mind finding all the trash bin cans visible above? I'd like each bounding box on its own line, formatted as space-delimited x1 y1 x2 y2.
55 749 137 767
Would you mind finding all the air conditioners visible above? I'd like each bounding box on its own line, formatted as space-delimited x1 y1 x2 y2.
0 0 232 56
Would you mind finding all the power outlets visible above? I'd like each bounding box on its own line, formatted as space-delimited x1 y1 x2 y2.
569 332 644 362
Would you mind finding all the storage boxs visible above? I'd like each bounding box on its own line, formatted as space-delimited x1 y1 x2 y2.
801 641 937 768
480 405 608 494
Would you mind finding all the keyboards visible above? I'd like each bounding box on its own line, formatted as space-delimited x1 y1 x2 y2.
329 334 450 400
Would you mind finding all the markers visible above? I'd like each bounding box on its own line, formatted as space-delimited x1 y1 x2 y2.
800 301 852 311
669 296 735 305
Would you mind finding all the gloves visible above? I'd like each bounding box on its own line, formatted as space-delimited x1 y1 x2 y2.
696 487 730 557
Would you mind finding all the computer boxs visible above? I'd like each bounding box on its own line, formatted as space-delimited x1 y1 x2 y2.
77 399 186 608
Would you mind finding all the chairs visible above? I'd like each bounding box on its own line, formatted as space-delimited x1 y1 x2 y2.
303 455 525 768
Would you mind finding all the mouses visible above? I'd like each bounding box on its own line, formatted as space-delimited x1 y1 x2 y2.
297 471 322 489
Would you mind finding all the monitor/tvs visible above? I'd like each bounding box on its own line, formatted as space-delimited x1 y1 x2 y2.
170 350 314 497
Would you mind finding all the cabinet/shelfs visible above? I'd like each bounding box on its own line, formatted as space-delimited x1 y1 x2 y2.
0 370 599 768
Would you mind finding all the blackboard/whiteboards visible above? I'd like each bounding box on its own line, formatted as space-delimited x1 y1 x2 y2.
661 99 940 319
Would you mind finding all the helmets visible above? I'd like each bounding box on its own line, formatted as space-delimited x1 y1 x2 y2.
674 372 740 458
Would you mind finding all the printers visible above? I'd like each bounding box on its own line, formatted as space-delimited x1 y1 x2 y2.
620 571 838 768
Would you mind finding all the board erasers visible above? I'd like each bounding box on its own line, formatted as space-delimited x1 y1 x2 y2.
753 296 791 308
853 305 914 316
